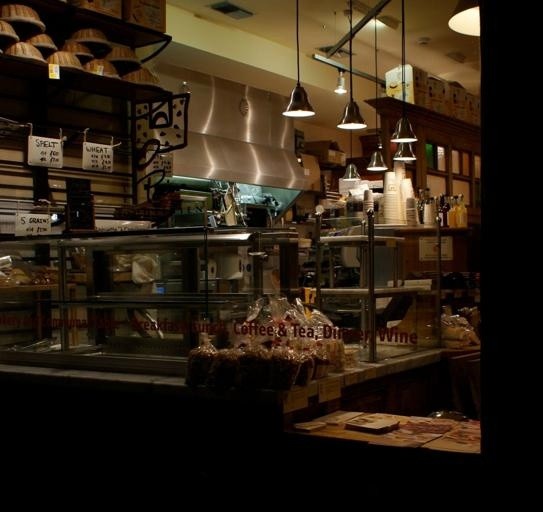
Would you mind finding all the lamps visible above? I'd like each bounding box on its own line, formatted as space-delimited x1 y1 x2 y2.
342 129 362 183
282 0 315 118
447 0 481 37
366 14 388 171
336 0 368 130
389 0 419 161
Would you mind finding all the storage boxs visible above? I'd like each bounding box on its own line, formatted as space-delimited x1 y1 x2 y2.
304 141 346 168
383 64 480 125
69 0 166 32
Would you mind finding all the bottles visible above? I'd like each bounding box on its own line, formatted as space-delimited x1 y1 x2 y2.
417 188 468 229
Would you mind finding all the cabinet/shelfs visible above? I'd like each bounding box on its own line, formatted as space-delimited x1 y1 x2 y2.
1 1 172 118
365 97 481 224
0 206 444 376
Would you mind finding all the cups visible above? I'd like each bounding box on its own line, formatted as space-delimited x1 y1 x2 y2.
362 159 419 225
